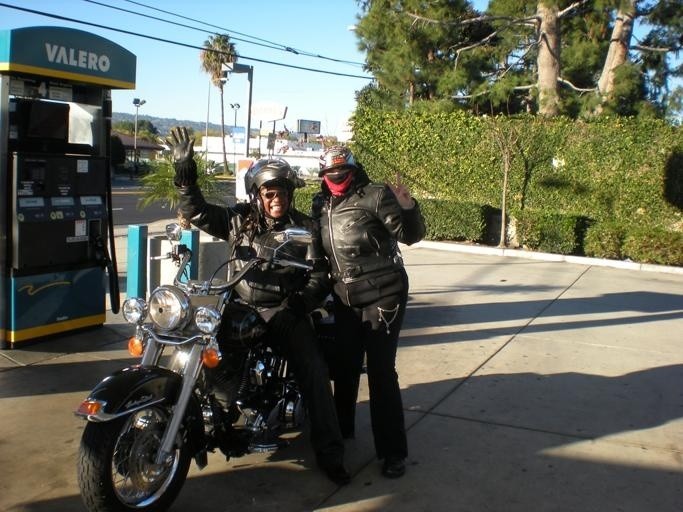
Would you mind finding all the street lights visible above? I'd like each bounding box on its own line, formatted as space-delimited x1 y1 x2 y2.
229 103 240 128
132 97 146 177
221 61 253 158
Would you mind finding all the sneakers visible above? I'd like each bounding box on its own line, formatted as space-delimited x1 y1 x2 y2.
317 457 350 485
384 459 404 477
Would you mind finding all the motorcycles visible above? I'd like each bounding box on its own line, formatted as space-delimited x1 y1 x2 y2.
70 218 368 512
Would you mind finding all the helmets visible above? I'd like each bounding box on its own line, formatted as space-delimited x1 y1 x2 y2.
246 160 299 201
318 146 359 177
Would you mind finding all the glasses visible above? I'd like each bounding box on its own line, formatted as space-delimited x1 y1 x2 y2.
260 191 286 199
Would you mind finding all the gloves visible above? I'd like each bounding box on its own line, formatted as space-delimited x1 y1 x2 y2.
259 306 285 322
164 126 193 162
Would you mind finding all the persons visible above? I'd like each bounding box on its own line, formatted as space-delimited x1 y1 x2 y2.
309 144 427 477
163 127 353 489
